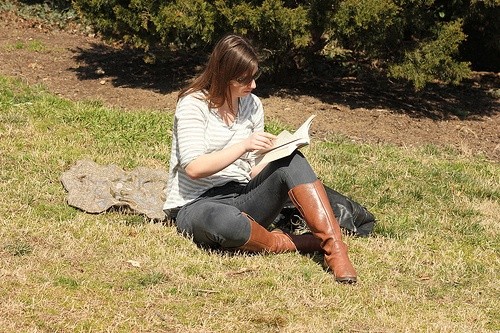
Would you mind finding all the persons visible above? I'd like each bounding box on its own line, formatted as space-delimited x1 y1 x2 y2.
163 33 357 282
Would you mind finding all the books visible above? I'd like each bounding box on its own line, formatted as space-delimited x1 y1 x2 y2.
255 114 317 165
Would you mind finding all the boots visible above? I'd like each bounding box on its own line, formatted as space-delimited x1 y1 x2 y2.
237 212 327 254
288 178 358 284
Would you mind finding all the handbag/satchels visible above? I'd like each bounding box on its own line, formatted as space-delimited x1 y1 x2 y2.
322 184 375 238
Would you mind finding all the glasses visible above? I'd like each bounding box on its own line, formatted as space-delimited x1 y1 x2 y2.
236 65 262 85
